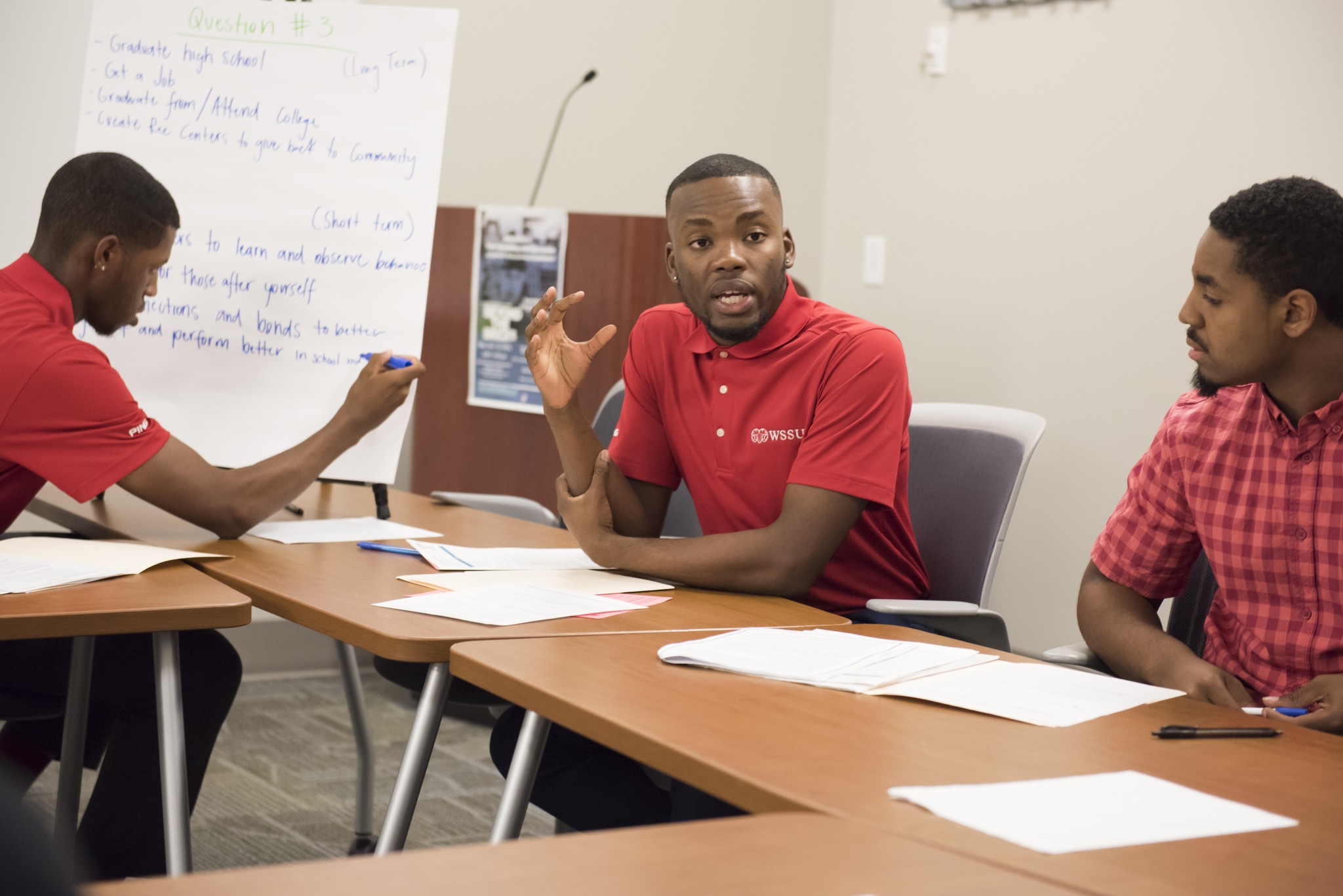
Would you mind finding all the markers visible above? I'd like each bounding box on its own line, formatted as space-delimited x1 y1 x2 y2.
360 352 411 368
1240 706 1307 716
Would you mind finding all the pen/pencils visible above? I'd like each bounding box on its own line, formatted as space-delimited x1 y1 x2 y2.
1151 724 1284 739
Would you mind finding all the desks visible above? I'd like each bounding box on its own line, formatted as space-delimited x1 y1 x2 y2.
23 469 851 856
72 810 1111 896
0 535 251 879
444 622 1341 896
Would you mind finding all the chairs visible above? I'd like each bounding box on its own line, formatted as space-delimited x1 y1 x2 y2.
551 401 1048 834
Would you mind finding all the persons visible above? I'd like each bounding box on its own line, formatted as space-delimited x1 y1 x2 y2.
1075 177 1343 742
0 153 430 884
488 151 932 834
